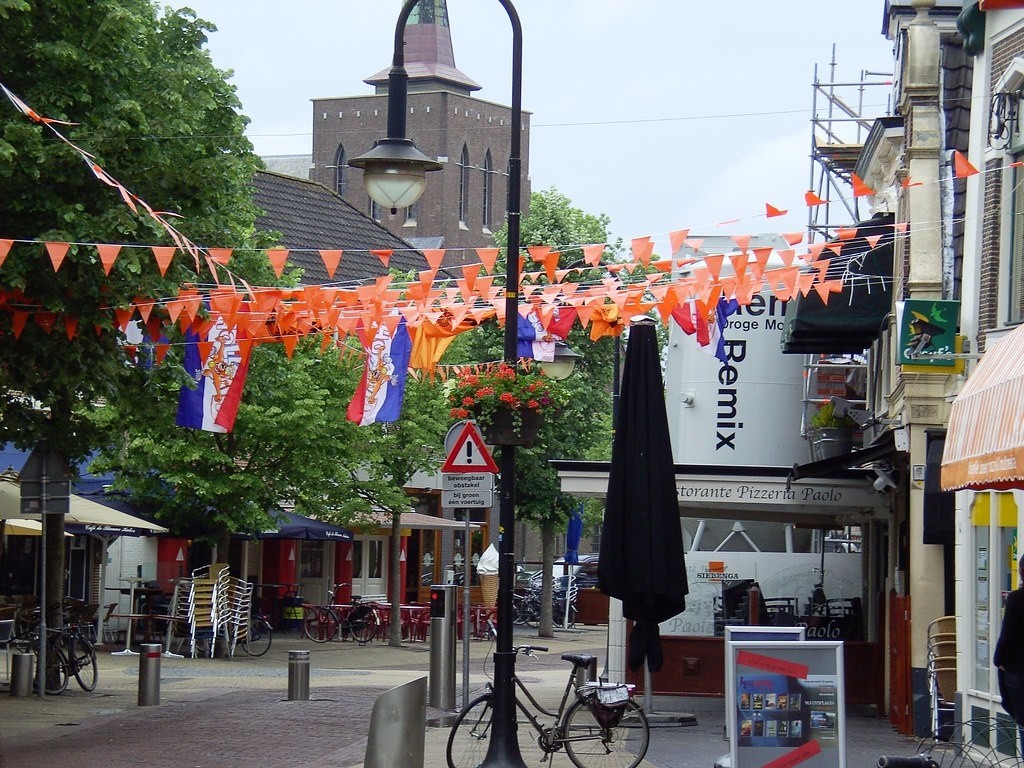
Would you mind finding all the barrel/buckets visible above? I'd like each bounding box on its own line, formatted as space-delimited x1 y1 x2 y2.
279 598 304 631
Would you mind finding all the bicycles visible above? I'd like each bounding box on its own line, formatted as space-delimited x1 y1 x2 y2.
239 598 275 658
303 582 381 646
444 618 651 768
22 599 99 695
523 579 579 628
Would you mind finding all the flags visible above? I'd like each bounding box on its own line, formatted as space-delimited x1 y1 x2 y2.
175 302 254 434
346 307 411 426
409 308 497 369
516 308 577 362
671 297 739 367
118 320 170 368
589 303 657 341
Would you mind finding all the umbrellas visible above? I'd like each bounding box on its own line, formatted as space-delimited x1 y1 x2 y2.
596 314 690 713
0 464 352 649
563 504 583 629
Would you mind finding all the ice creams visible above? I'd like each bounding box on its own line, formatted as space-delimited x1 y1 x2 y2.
476 542 499 621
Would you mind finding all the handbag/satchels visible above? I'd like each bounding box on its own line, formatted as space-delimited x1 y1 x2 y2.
592 684 630 728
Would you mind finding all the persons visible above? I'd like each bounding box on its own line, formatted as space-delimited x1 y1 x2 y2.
992 555 1024 759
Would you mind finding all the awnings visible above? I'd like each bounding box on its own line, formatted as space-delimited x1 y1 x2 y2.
284 508 480 531
940 322 1024 492
780 218 895 354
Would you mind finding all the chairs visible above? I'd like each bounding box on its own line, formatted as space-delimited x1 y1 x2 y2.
299 596 499 654
63 600 85 633
99 602 118 643
83 602 101 642
0 606 20 649
0 619 16 680
165 557 255 660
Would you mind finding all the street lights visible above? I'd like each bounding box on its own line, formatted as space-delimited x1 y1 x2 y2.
346 1 529 768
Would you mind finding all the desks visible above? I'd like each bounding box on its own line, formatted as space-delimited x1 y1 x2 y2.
108 612 151 656
471 604 497 636
154 614 189 660
377 602 407 606
323 605 361 642
104 587 164 644
400 604 428 641
